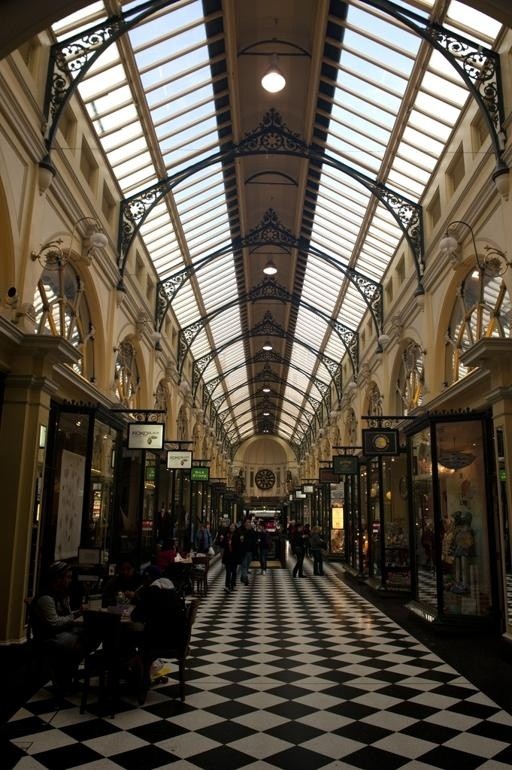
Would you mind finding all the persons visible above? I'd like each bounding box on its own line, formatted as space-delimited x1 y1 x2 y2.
37 555 189 693
157 513 327 592
450 501 476 594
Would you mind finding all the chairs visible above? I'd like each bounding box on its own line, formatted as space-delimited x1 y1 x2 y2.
30 552 209 713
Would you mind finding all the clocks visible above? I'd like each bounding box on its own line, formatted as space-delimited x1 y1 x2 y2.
256 468 276 489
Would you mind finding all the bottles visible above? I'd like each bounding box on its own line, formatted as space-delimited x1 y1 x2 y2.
88 594 102 612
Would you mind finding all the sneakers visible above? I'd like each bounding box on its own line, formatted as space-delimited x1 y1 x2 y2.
293 569 324 576
224 576 248 592
259 569 265 575
